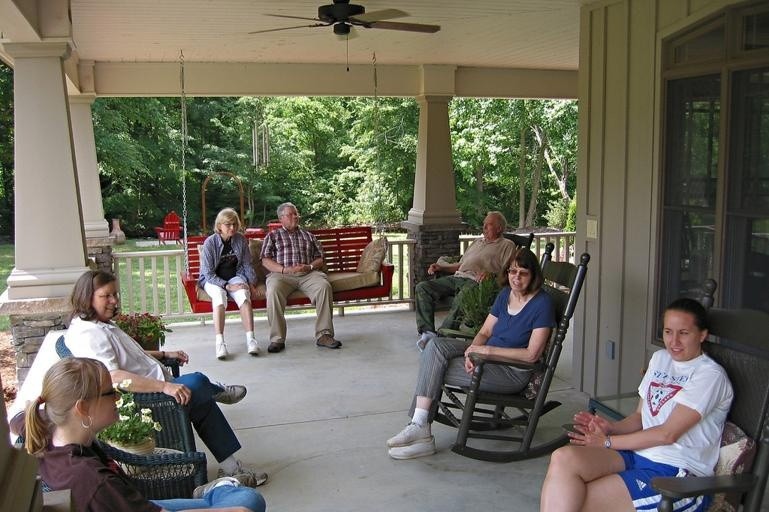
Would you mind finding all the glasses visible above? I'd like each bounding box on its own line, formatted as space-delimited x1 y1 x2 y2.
507 269 529 276
98 291 120 301
64 389 122 414
282 213 299 218
223 222 237 227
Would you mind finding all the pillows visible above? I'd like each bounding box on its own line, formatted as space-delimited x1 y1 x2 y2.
356 236 389 273
710 421 756 512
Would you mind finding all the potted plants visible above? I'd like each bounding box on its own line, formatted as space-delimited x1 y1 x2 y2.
115 311 171 351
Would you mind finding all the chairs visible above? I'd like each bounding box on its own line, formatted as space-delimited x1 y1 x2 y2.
562 279 769 512
428 243 590 463
15 435 207 512
154 211 183 246
434 233 536 328
55 335 196 452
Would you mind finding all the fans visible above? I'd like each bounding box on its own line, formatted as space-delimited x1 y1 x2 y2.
247 0 440 35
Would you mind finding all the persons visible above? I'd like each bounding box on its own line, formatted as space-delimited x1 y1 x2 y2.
198 207 259 360
24 358 266 512
63 270 268 487
414 212 517 349
387 248 557 460
540 297 734 512
259 202 342 353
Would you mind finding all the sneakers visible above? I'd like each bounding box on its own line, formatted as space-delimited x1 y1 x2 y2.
268 342 285 353
247 337 259 354
211 381 247 404
192 474 257 499
388 435 437 460
216 342 228 358
416 331 437 352
317 334 342 348
386 421 432 447
217 459 268 487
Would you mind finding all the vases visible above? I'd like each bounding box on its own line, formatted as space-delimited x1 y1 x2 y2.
105 438 154 475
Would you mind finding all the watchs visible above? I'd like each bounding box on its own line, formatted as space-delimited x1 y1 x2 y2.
604 436 611 449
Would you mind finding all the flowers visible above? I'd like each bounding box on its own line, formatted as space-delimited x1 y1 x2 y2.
98 379 162 444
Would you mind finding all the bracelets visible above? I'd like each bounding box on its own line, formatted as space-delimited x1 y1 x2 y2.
162 350 166 357
281 266 283 274
310 264 314 271
